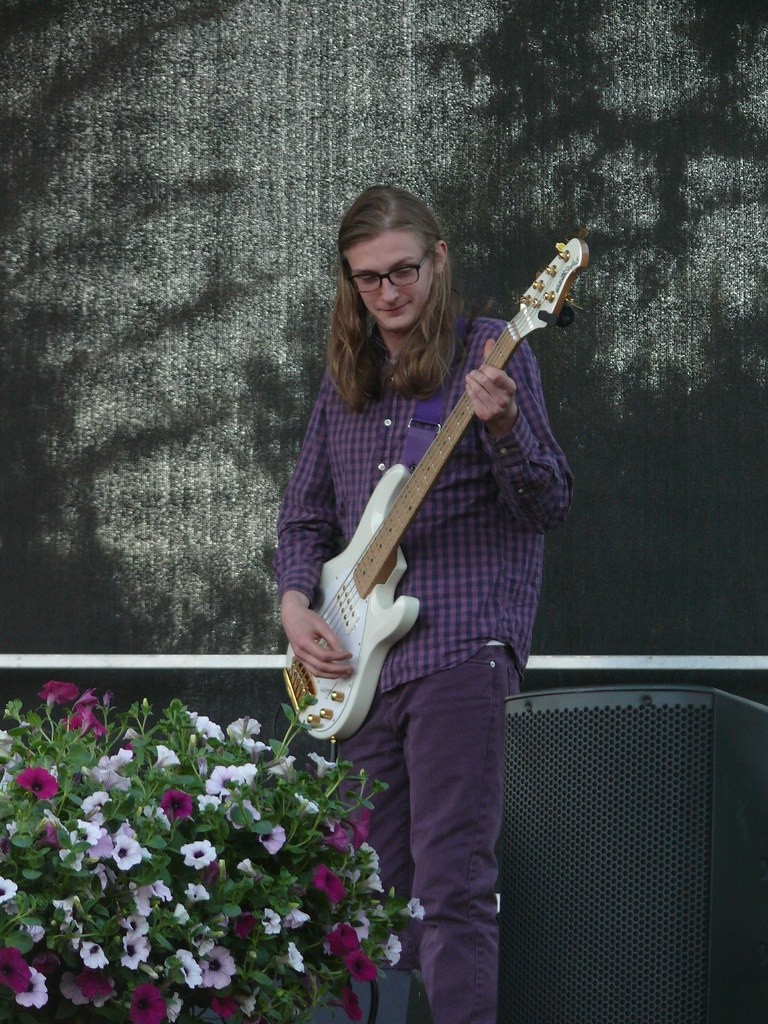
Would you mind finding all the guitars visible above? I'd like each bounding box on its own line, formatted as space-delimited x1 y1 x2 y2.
278 229 589 743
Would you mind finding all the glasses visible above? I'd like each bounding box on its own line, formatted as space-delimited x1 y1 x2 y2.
347 238 438 293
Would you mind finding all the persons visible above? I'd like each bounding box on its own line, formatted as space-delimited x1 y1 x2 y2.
273 185 575 1024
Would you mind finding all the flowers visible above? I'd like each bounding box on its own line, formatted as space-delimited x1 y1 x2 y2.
0 679 425 1024
